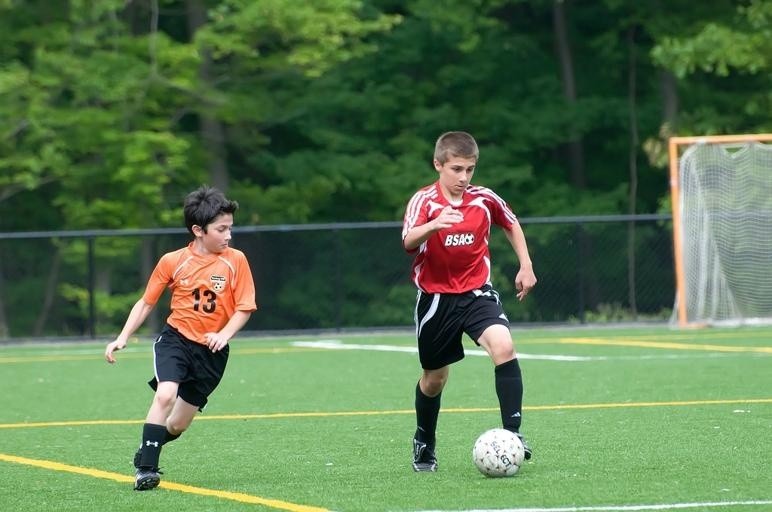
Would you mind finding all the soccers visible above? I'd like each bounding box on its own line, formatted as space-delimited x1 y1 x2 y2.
472 428 524 476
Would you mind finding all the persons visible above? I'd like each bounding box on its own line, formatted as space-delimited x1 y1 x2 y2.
102 184 258 491
400 129 539 474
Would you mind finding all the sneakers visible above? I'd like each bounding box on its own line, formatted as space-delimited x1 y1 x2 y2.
133 442 144 464
412 432 437 473
514 431 532 459
133 467 163 491
